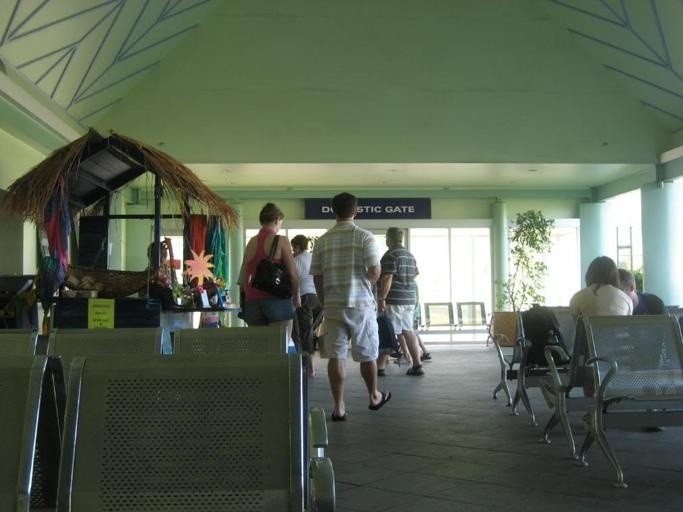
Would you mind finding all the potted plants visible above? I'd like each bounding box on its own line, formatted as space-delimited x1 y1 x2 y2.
487 206 557 348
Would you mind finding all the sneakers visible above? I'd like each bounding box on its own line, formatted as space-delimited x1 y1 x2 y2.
394 355 411 364
377 368 385 377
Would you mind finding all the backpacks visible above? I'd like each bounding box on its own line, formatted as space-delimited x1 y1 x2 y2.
377 311 399 354
521 303 570 367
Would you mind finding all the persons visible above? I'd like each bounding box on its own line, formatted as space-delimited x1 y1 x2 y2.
615 269 669 433
569 256 634 384
139 237 180 303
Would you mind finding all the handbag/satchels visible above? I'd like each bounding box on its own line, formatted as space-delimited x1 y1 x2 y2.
252 257 293 299
317 331 331 359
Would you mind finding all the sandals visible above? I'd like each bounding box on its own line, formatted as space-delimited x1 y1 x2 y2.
420 352 432 361
406 365 424 376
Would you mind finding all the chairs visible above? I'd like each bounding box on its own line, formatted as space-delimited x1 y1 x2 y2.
174 326 328 458
44 327 164 356
487 305 683 490
0 324 338 512
456 300 490 335
0 355 61 511
0 330 38 356
55 355 335 512
423 301 457 334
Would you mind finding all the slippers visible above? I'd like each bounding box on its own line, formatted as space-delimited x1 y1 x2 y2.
331 410 346 422
368 390 391 410
